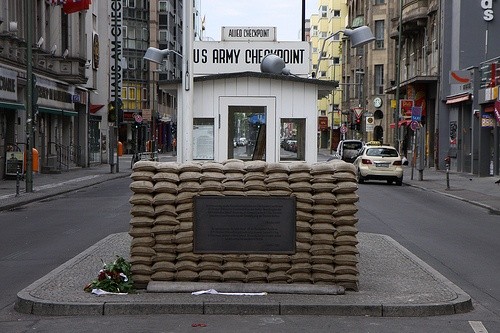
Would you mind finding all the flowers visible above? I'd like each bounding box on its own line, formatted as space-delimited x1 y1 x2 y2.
83 255 136 295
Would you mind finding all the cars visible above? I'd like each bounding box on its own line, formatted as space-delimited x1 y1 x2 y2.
232 134 298 154
353 146 403 186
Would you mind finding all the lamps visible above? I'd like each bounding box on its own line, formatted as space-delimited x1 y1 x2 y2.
36 37 44 48
62 49 68 58
143 46 169 64
10 22 18 32
260 54 291 75
344 25 376 48
50 44 57 55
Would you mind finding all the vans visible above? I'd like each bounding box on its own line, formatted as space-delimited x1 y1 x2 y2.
335 139 363 163
